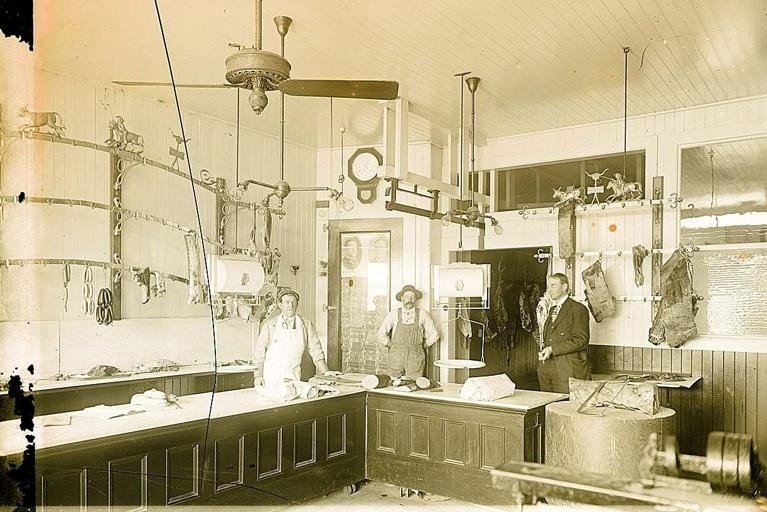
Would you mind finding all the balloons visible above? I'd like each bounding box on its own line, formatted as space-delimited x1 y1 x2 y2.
344 148 383 205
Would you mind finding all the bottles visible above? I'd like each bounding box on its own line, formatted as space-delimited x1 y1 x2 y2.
396 285 422 301
277 290 299 300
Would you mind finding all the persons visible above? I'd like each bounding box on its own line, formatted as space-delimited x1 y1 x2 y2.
377 284 438 382
536 273 591 393
343 239 360 269
253 290 340 386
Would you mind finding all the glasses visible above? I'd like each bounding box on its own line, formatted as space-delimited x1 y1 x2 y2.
442 70 504 237
223 15 354 219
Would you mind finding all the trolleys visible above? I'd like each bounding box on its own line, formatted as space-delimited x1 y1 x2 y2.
111 1 402 120
